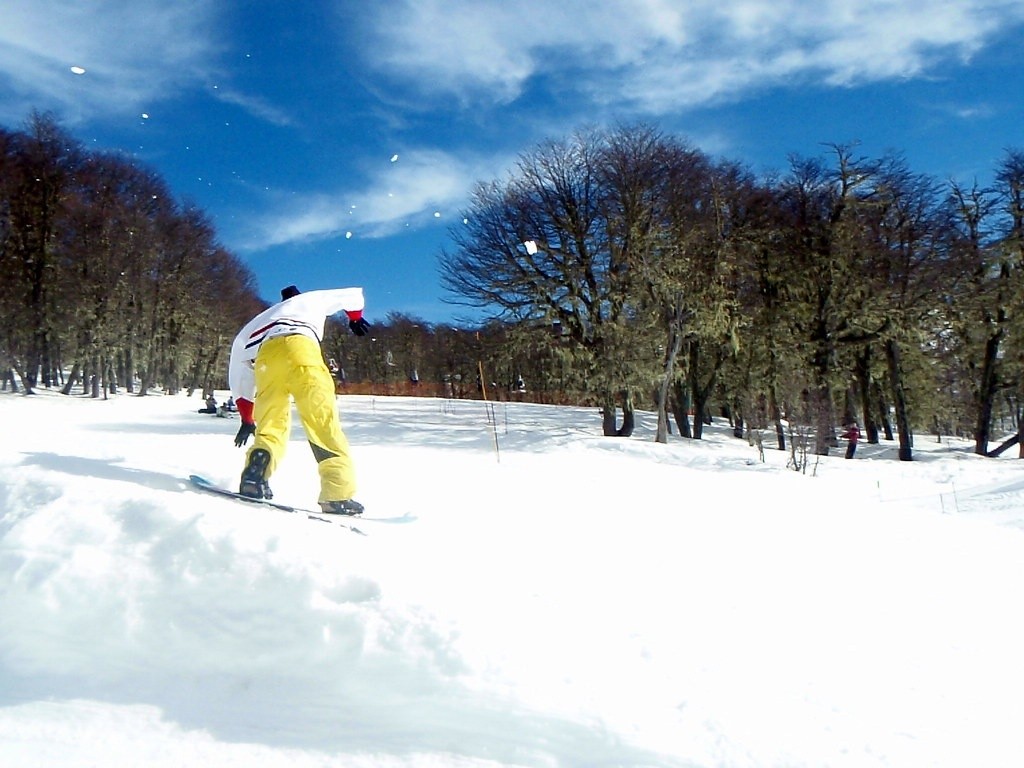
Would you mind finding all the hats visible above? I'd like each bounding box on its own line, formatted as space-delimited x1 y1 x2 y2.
282 286 301 300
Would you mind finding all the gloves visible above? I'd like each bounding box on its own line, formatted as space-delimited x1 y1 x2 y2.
349 318 371 337
234 420 257 448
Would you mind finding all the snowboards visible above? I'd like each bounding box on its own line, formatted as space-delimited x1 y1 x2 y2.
188 470 415 533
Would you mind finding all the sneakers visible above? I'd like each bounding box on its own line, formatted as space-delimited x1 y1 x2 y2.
240 448 274 500
321 499 364 516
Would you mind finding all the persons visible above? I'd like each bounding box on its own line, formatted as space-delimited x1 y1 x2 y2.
227 396 236 406
229 285 364 516
205 394 217 409
839 422 860 459
219 403 237 413
